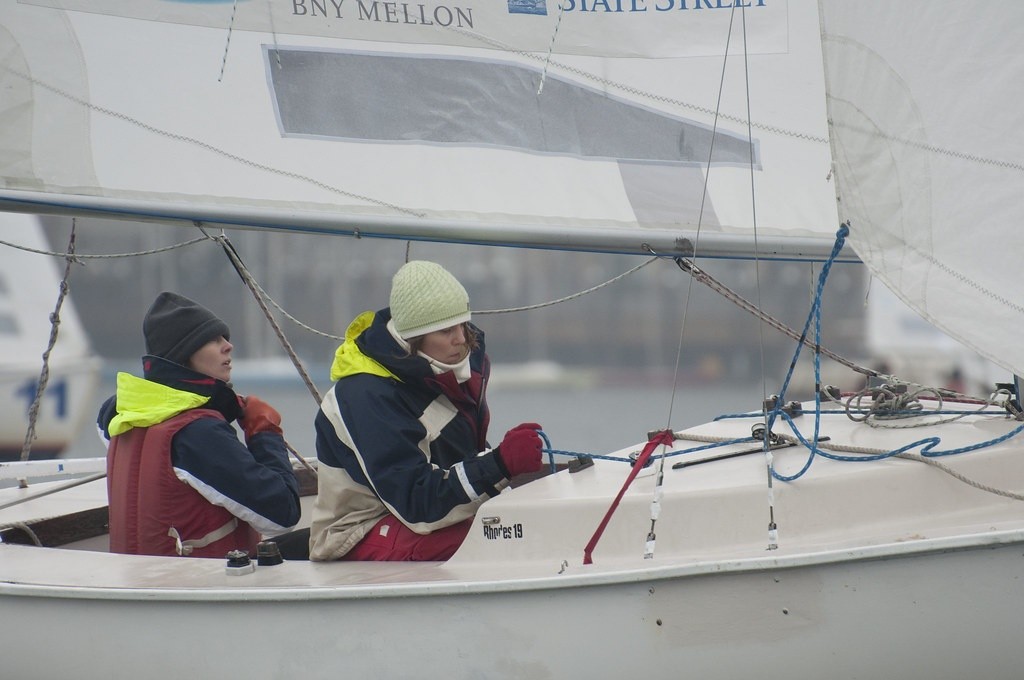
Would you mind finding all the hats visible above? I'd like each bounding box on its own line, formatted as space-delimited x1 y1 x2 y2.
141 292 231 363
388 259 471 341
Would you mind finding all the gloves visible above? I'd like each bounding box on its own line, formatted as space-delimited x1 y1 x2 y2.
235 394 284 444
492 422 544 479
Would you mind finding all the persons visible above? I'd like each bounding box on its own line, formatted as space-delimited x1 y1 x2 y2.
310 257 544 563
97 288 304 560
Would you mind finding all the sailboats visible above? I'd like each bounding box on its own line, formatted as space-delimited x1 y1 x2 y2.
0 0 1024 678
